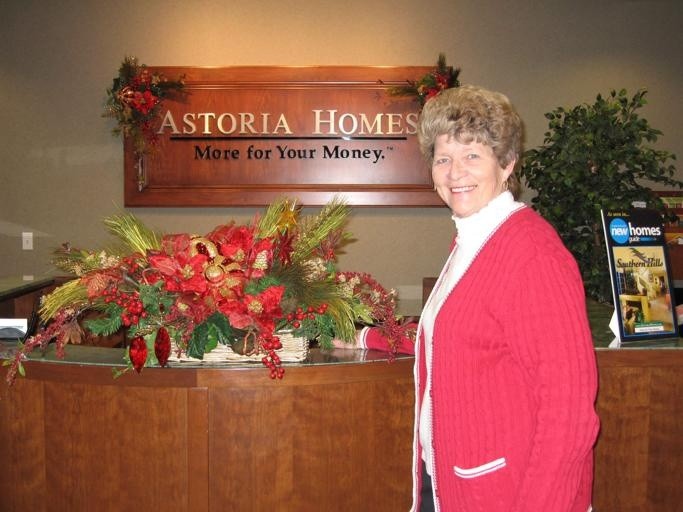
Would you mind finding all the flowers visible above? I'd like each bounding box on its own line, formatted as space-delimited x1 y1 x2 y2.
386 53 462 120
0 195 417 387
101 56 192 185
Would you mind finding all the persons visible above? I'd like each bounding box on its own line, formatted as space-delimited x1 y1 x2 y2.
407 88 601 512
